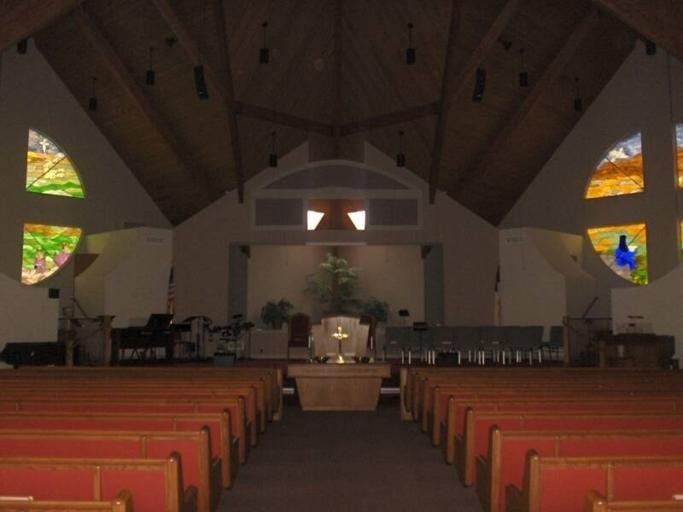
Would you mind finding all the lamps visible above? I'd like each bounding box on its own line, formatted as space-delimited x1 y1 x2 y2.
406 22 416 66
396 129 405 168
259 20 270 64
471 64 488 102
146 45 155 86
88 76 99 112
518 46 528 89
18 36 27 55
192 64 208 102
646 39 657 57
268 129 278 167
573 77 583 112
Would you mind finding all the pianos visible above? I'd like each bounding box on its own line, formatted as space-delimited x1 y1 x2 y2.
110 328 176 364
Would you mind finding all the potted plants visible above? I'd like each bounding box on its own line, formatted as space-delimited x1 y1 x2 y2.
261 297 294 330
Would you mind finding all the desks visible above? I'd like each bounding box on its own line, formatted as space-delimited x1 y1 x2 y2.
75 315 116 365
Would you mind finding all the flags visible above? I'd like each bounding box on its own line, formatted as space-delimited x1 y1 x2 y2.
167 268 176 310
494 268 501 321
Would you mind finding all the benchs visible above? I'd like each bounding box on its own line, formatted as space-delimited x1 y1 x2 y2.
400 365 683 512
0 366 283 512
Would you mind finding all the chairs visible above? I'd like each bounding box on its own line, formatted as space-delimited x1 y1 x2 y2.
381 325 564 364
111 313 174 362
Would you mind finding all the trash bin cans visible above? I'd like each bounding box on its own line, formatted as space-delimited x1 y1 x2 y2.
215 350 233 367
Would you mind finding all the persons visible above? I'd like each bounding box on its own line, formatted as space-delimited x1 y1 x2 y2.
611 234 638 273
